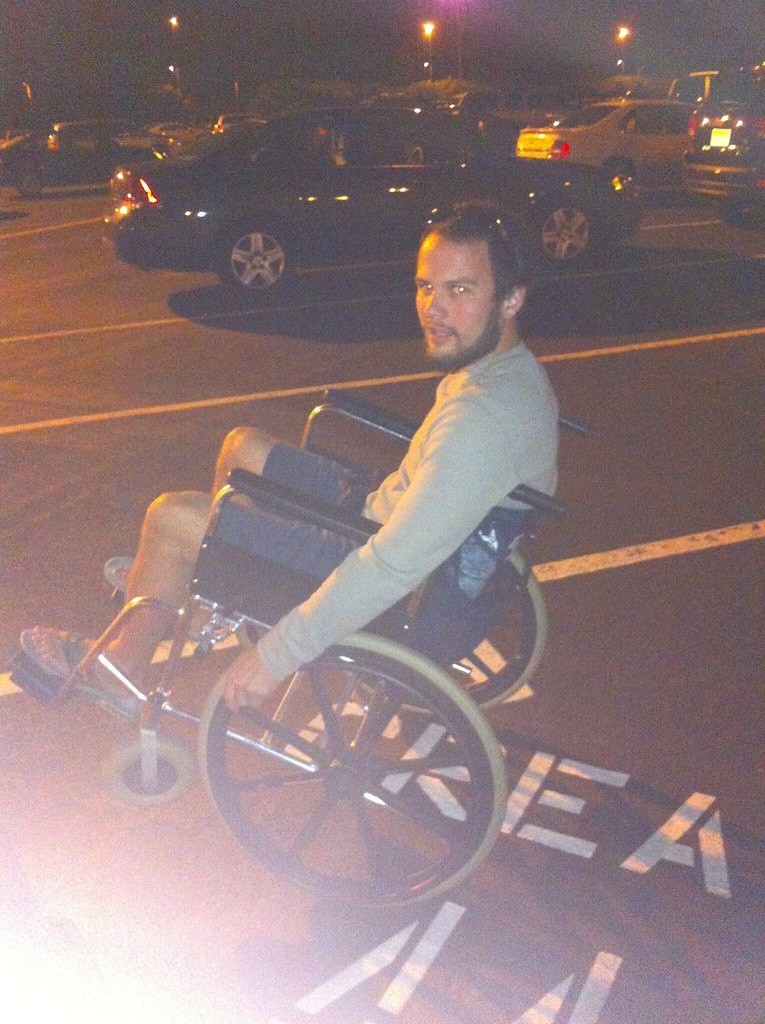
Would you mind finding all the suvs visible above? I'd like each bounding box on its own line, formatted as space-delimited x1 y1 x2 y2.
680 59 762 236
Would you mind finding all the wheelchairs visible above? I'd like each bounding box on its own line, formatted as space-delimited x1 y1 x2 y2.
8 387 561 910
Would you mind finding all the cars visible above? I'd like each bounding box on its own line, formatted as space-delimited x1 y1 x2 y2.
514 100 693 184
102 106 641 298
0 118 170 200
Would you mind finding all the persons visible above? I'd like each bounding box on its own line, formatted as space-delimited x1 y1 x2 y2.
20 200 561 721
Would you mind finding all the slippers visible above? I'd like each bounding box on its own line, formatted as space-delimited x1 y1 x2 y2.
21 626 140 722
104 557 136 594
